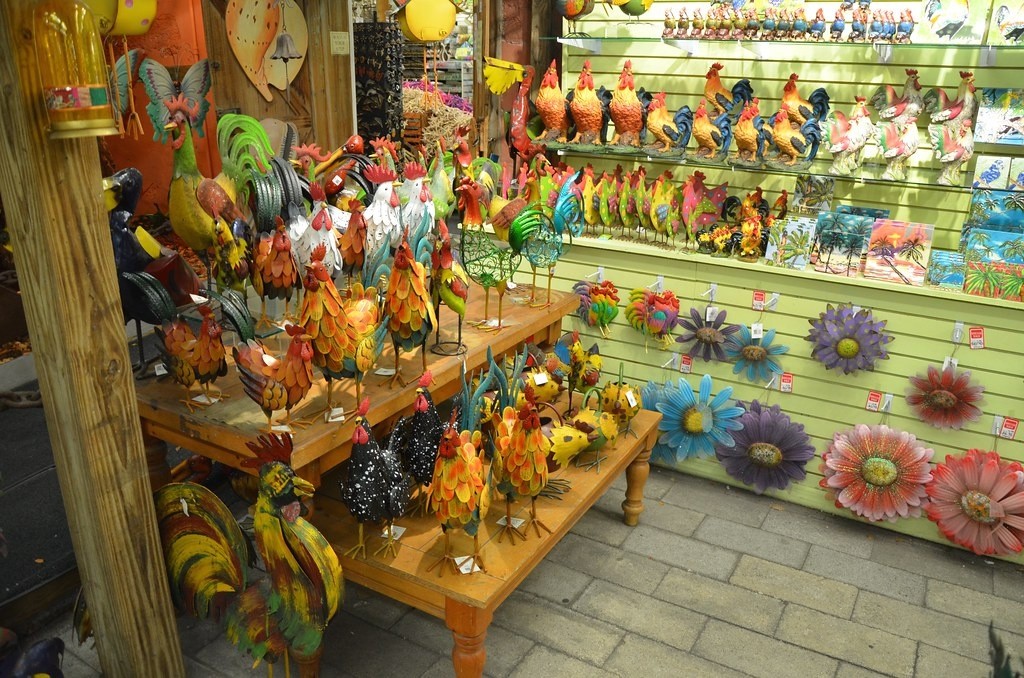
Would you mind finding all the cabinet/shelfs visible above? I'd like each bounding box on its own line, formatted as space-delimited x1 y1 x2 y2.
459 37 1024 311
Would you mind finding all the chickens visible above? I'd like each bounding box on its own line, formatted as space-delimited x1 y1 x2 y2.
0 58 1022 678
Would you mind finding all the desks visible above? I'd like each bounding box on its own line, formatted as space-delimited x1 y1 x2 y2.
313 390 663 678
135 275 581 498
